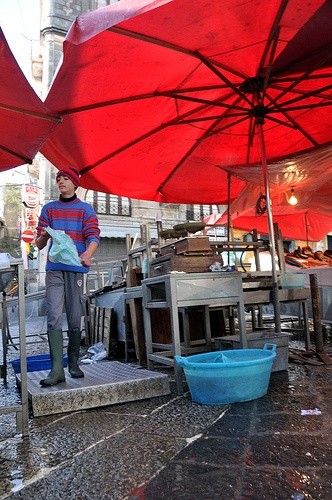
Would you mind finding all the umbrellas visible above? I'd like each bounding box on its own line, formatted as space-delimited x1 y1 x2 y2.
202 140 332 254
0 26 63 174
37 0 332 332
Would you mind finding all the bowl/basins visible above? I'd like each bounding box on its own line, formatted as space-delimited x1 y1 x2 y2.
7 354 71 374
280 271 306 289
175 343 278 405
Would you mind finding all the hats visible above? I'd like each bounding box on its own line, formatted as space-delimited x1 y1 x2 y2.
56 167 79 187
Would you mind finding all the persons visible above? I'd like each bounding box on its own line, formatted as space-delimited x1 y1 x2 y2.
34 167 101 387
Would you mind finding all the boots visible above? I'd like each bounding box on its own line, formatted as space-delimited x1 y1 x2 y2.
67 330 84 378
40 329 65 386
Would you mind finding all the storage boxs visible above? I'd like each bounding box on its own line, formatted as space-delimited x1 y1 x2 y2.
213 332 293 372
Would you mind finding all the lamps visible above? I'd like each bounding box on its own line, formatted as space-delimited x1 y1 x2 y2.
287 186 298 205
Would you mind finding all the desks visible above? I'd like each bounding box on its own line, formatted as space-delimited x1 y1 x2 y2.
124 221 311 395
